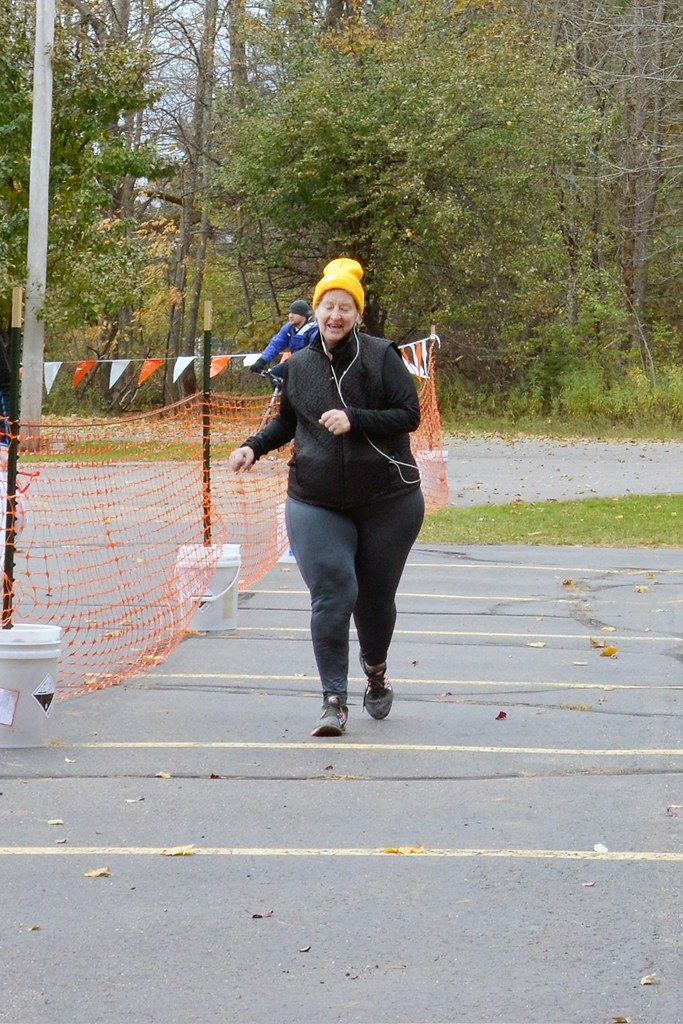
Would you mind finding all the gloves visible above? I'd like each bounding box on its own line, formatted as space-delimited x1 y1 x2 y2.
249 360 265 373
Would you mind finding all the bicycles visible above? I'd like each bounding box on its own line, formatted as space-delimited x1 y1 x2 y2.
253 369 284 434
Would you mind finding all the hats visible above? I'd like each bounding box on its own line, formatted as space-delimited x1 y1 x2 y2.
312 257 365 316
288 300 312 316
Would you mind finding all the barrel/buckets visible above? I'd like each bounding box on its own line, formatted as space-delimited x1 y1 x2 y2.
0 624 62 747
276 504 297 563
174 544 241 631
416 450 448 512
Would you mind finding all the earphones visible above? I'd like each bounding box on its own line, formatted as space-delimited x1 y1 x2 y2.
354 313 360 327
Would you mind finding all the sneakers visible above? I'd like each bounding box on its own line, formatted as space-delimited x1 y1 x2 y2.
311 694 348 736
359 648 394 719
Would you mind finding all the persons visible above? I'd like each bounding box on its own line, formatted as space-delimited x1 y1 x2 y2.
228 258 425 737
250 300 319 395
